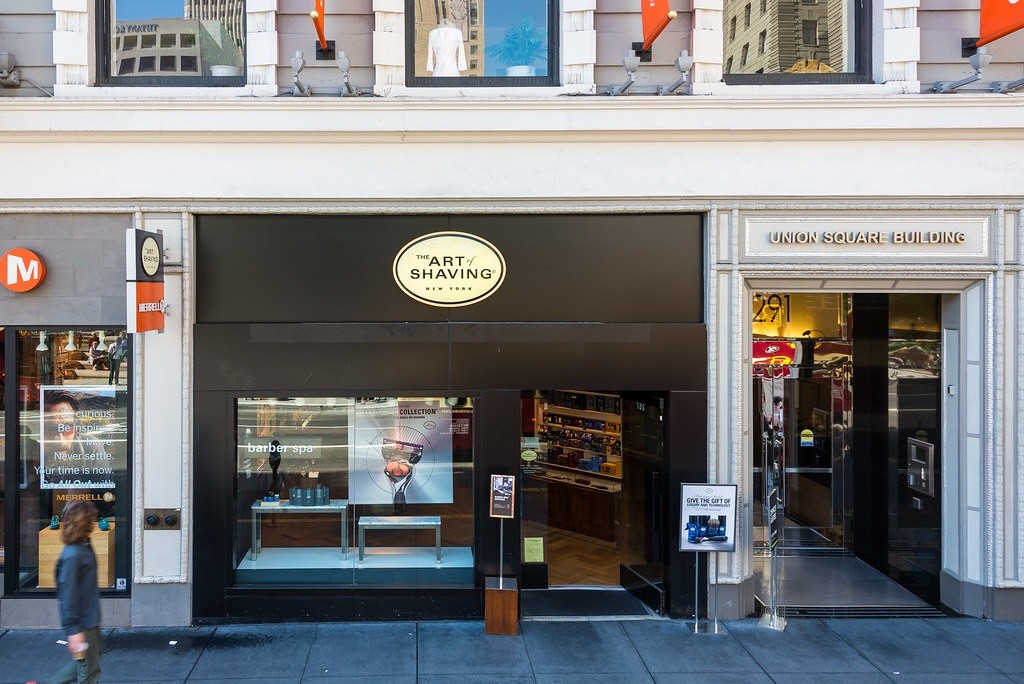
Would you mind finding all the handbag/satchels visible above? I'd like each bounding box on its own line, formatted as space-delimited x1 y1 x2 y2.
109 352 115 361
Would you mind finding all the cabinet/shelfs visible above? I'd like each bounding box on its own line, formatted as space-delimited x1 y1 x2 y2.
38 521 115 588
521 390 623 541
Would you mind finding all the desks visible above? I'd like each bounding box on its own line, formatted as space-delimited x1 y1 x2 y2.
252 499 350 560
358 516 441 564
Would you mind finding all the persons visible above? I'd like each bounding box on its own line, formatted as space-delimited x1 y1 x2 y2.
427 19 466 77
48 498 102 684
89 332 126 384
44 391 112 481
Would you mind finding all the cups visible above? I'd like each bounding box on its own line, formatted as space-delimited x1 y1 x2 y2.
263 497 273 502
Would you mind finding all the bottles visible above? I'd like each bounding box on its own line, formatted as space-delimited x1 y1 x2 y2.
274 494 279 502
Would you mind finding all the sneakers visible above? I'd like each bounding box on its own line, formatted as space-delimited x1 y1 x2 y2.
50 515 59 529
99 518 110 531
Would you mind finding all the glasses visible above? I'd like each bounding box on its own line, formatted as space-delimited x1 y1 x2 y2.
44 424 77 436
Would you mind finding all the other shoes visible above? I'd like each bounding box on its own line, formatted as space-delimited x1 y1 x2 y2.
26 681 38 684
109 382 112 385
93 366 97 371
104 367 109 370
115 383 121 386
